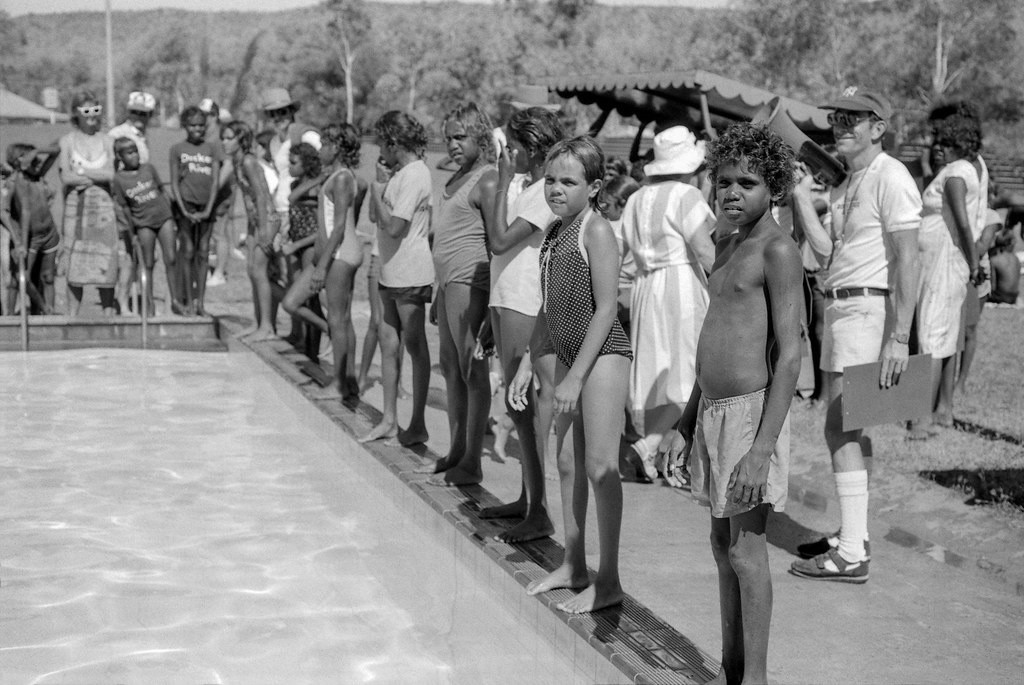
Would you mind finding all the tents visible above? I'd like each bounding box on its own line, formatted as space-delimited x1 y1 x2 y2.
0 88 71 124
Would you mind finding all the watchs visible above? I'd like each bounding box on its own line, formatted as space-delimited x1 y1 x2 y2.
890 332 909 344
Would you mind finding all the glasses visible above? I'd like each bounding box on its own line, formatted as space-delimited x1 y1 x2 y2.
76 104 104 117
827 112 870 129
269 109 287 118
130 110 148 116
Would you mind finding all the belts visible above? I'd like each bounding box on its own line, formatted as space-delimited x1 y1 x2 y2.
823 286 888 299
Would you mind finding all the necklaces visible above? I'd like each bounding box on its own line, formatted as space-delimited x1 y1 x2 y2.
834 151 883 249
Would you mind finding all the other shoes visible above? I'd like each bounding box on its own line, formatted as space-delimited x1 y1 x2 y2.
790 536 872 584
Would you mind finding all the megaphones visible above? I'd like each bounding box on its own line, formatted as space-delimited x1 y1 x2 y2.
752 96 848 188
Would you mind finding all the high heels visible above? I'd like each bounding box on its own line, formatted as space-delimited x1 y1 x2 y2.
625 438 659 482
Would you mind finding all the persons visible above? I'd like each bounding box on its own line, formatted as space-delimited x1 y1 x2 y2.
791 84 1024 583
507 136 634 614
358 110 435 448
0 88 718 544
663 123 805 684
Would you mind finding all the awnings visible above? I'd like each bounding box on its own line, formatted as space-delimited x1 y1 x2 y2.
548 70 895 150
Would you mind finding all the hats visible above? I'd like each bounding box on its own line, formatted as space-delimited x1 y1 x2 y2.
126 91 156 112
642 124 707 177
260 87 301 115
818 84 894 121
198 98 219 113
